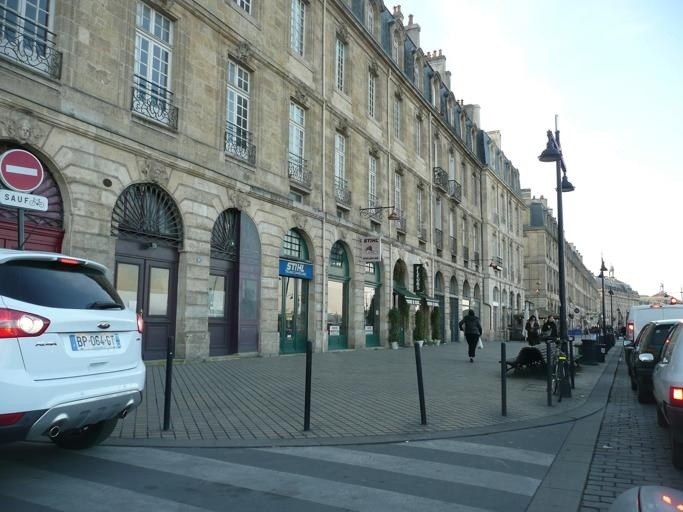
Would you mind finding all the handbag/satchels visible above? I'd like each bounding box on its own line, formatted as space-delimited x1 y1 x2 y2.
542 329 552 338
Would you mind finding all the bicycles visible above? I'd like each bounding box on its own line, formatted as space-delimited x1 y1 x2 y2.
544 334 576 405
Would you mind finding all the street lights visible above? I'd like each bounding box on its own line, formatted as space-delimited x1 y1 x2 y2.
597 252 608 339
536 113 576 381
615 306 625 333
608 286 615 333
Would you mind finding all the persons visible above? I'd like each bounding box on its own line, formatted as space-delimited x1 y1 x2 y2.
525 314 557 346
459 310 482 363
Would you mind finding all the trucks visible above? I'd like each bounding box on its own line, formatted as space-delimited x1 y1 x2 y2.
623 303 683 380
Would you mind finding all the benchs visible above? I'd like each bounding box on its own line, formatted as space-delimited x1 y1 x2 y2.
498 342 542 375
533 342 582 373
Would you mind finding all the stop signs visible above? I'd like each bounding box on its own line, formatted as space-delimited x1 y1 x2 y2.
0 147 45 193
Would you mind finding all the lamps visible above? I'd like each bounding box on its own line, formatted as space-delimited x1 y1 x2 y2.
523 278 541 285
361 205 399 220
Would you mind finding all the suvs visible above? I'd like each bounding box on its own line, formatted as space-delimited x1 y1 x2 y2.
624 317 679 406
0 245 149 458
637 318 682 471
